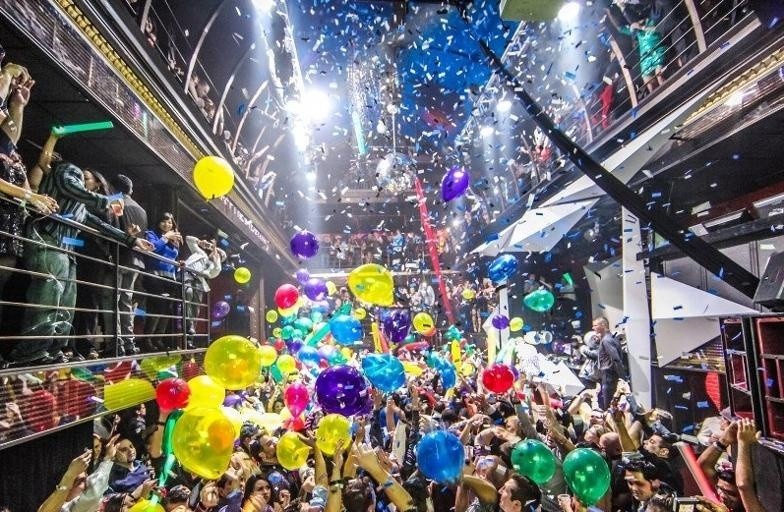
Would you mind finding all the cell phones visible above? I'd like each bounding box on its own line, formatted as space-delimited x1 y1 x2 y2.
111 203 123 216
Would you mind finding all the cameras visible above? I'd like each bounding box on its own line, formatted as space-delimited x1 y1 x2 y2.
204 243 211 249
673 497 701 512
